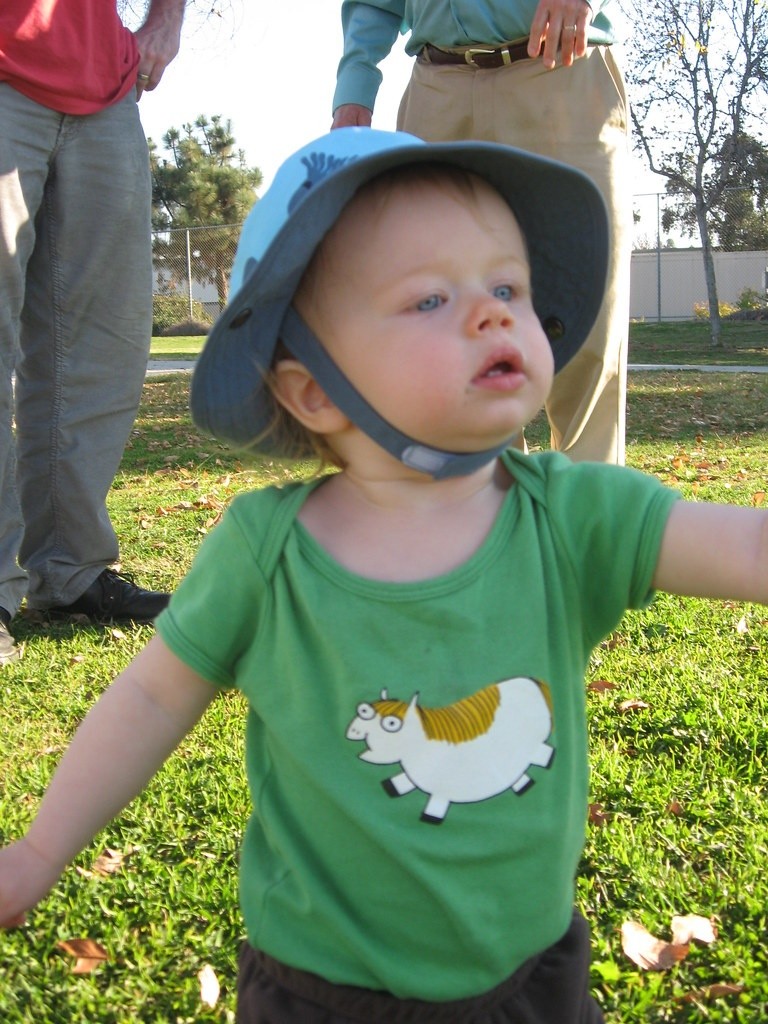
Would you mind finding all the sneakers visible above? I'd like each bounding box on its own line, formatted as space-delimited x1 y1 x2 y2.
0 607 19 663
28 569 172 623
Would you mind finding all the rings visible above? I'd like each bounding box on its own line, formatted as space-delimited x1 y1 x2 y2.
137 74 149 80
565 25 575 30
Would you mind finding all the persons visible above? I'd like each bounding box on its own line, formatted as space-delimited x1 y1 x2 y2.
0 128 767 1024
329 0 630 470
0 0 186 660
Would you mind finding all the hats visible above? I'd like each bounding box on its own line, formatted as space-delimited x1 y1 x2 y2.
193 124 608 461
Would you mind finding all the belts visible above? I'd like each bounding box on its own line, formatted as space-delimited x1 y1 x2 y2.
418 42 544 70
